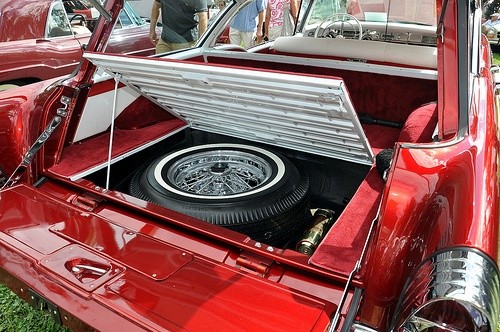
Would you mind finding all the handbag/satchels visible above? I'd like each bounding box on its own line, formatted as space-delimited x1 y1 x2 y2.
281 7 295 37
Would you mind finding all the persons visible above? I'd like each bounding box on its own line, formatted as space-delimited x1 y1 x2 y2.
149 0 208 54
481 13 500 35
217 0 298 49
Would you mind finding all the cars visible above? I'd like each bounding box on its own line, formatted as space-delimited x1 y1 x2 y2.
0 0 167 86
0 1 500 332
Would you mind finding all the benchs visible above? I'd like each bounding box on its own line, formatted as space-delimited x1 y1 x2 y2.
272 37 438 70
